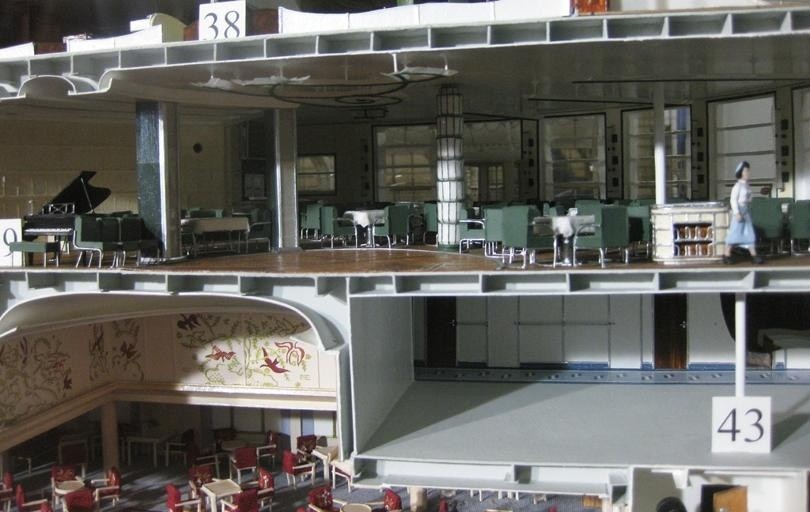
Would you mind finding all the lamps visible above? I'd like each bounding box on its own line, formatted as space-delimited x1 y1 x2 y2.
382 50 459 84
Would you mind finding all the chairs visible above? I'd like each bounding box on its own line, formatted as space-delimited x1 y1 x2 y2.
63 488 96 510
307 485 346 511
332 465 352 493
15 484 50 512
282 449 315 490
90 467 120 510
219 489 258 511
299 197 438 250
366 487 401 511
241 468 274 511
166 483 201 511
188 464 221 509
1 472 15 510
746 196 809 259
1 420 353 470
228 447 256 484
51 465 81 509
69 204 272 267
456 197 690 273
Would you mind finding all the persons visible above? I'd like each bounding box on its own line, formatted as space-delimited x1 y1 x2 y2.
722 160 764 264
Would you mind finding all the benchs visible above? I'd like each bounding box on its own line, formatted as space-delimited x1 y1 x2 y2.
10 240 60 267
